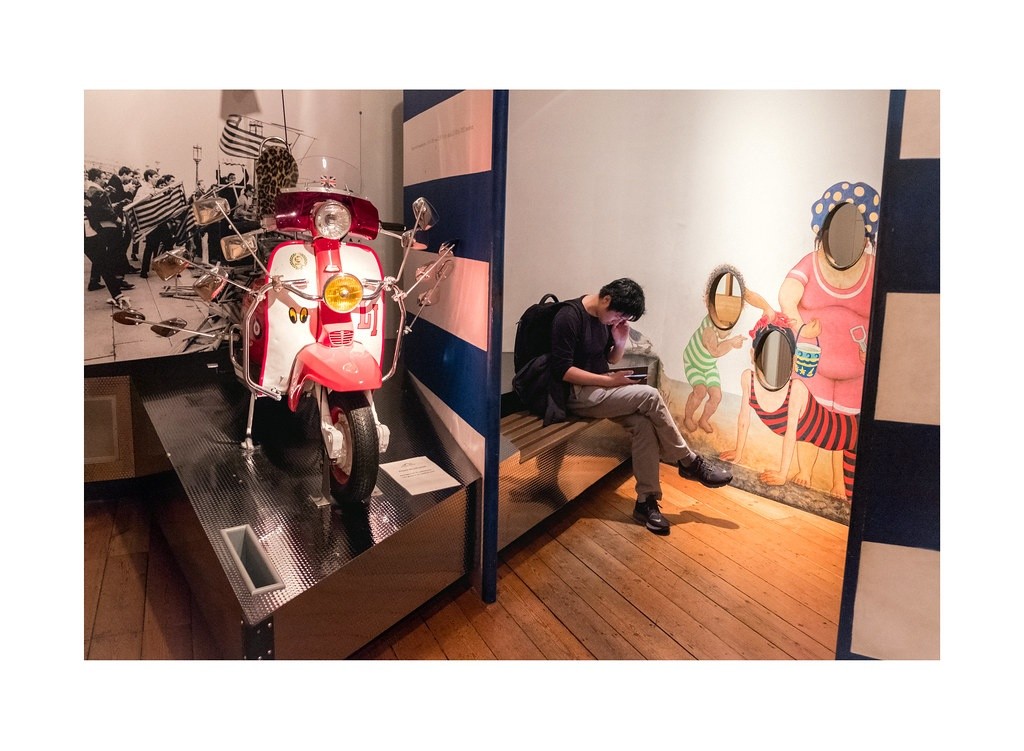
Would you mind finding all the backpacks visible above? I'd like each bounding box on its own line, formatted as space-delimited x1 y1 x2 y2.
513 292 586 376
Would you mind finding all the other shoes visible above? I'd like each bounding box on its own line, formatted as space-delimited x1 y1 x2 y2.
88 281 106 291
127 265 142 274
632 495 670 533
117 281 135 290
111 295 129 306
139 272 149 278
131 254 138 261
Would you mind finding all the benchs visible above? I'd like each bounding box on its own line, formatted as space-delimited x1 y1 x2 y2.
501 406 631 549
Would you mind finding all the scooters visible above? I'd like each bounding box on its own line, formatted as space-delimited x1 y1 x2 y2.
208 180 438 513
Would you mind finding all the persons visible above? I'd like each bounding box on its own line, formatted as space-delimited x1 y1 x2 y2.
85 167 248 300
548 277 732 532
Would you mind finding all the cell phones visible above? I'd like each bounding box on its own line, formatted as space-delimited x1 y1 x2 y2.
624 374 648 379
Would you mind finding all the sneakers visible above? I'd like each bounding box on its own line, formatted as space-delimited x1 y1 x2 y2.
678 454 733 489
